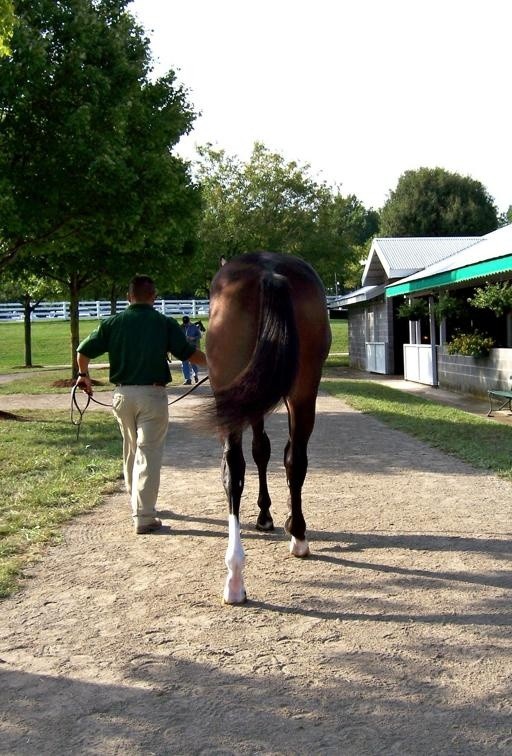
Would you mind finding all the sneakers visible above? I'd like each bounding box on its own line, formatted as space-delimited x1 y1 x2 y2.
136 517 161 534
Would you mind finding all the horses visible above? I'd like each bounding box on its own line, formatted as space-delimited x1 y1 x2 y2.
173 249 333 605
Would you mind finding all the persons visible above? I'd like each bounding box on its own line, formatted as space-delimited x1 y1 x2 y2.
180 316 202 385
75 276 207 534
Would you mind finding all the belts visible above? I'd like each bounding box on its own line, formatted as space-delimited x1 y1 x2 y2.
114 383 165 386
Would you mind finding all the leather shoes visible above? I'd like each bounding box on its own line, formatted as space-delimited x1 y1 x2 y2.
182 377 198 385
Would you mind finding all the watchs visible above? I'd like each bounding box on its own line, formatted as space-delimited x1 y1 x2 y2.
78 371 89 377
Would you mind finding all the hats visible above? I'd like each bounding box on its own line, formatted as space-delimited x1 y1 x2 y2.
183 317 190 323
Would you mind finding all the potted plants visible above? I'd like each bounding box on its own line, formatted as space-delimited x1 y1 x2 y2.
395 290 461 321
